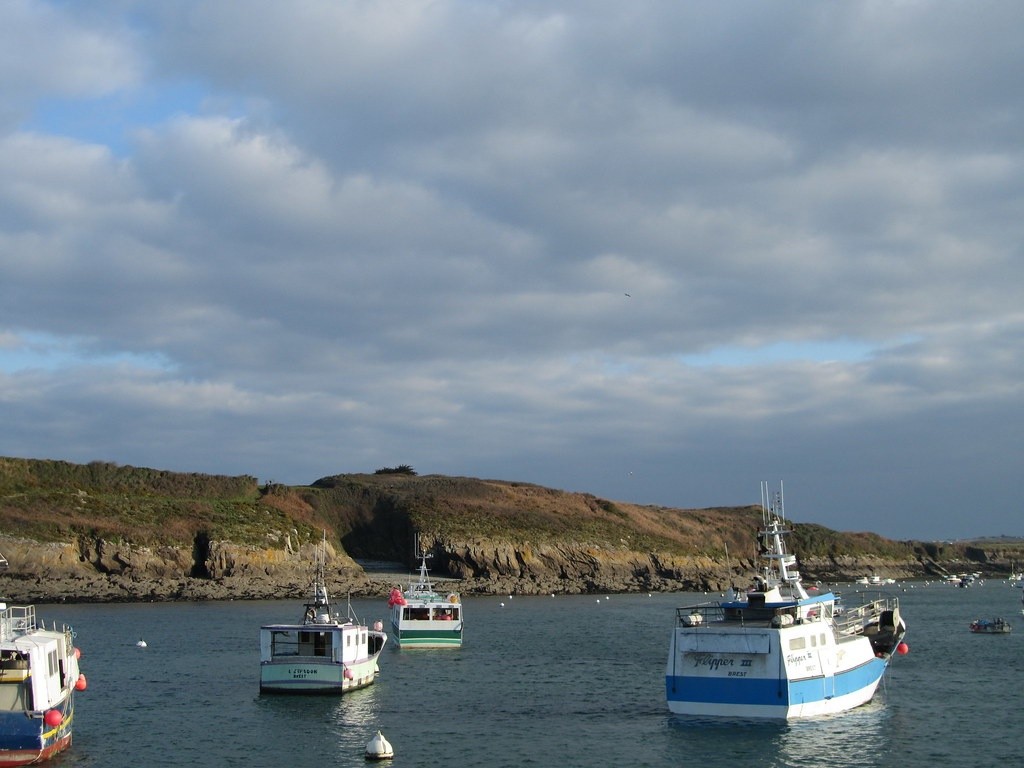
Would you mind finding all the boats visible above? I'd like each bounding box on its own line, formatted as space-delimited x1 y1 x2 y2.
856 577 868 584
390 532 464 649
970 617 1012 633
869 577 885 585
0 552 80 768
946 574 961 583
666 480 906 719
260 529 388 694
